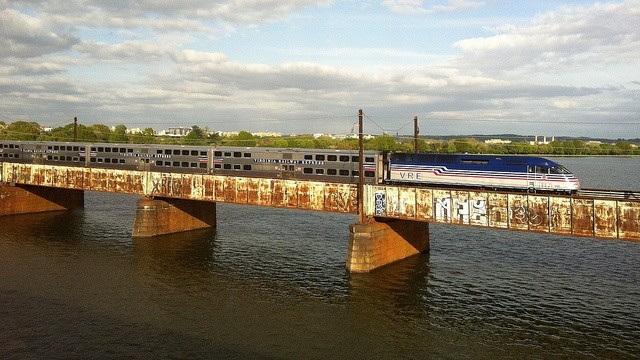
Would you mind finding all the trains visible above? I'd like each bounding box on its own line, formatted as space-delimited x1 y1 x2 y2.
0 139 580 196
